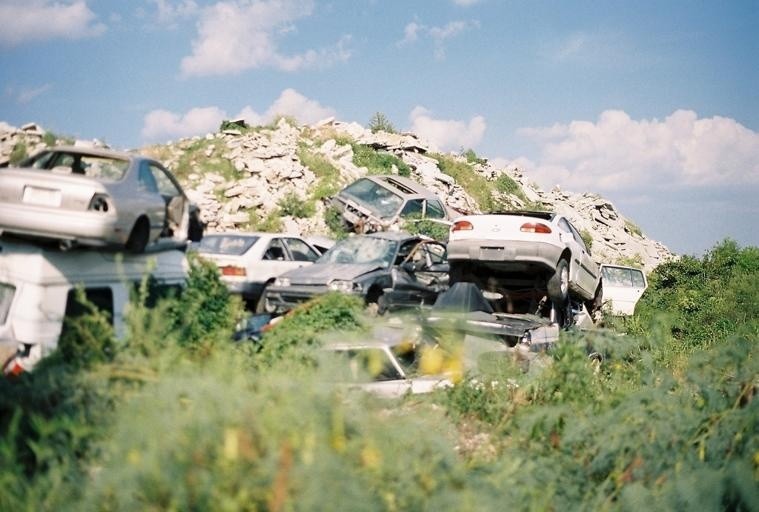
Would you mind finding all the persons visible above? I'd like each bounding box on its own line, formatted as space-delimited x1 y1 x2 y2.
189 204 211 241
